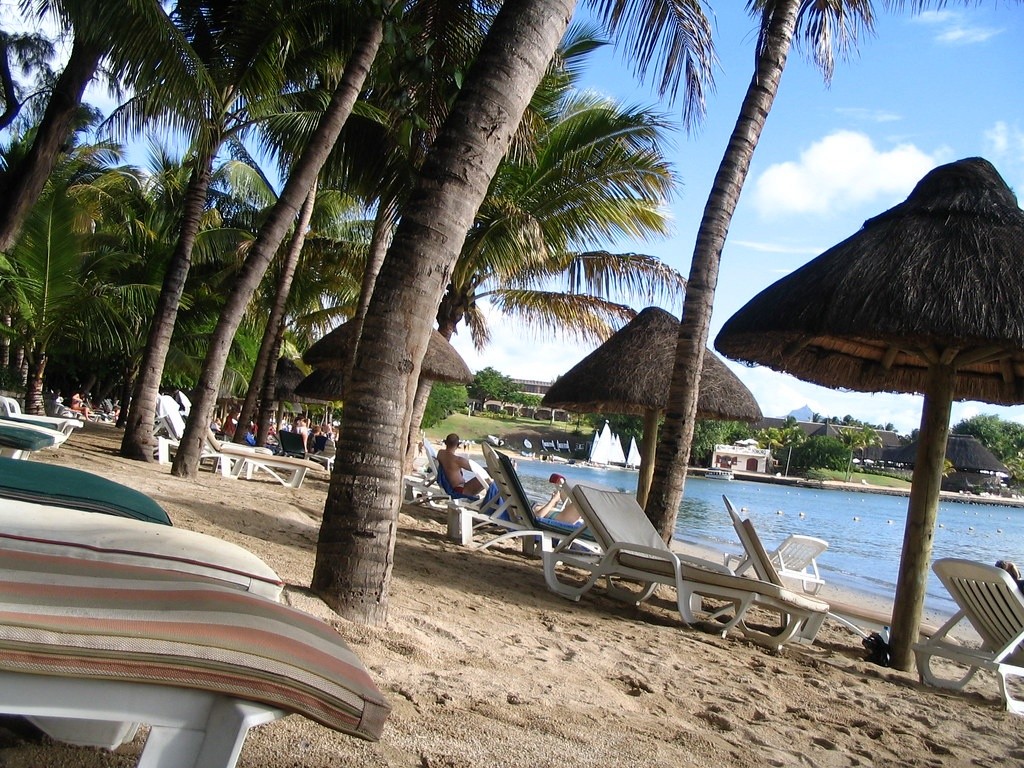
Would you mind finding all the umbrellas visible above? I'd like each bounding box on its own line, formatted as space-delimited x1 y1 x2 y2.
293 369 344 401
713 156 1024 671
303 308 474 383
274 356 328 434
541 306 762 512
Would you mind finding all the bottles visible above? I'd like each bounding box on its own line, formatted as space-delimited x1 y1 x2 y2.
880 626 890 660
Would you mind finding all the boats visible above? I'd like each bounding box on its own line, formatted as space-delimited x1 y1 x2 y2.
705 467 735 482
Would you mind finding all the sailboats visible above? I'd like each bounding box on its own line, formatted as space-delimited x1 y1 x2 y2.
577 418 642 473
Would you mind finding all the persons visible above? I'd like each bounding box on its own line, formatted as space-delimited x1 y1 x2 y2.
436 432 484 497
223 408 327 454
509 458 581 524
51 387 122 423
992 560 1024 612
